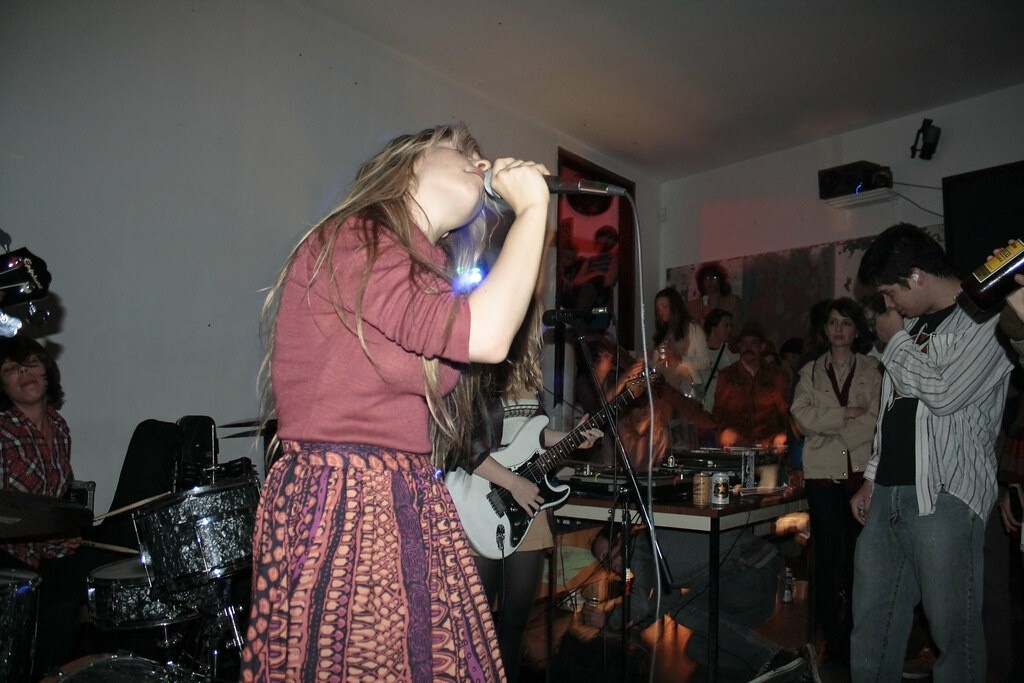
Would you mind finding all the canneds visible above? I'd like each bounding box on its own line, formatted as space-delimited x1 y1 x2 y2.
710 473 730 504
586 597 601 608
692 473 711 505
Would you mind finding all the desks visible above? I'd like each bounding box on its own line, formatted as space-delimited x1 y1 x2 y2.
547 492 816 683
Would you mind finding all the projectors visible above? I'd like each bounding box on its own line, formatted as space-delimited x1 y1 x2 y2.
817 161 894 199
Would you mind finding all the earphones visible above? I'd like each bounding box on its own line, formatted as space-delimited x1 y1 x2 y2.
911 274 919 282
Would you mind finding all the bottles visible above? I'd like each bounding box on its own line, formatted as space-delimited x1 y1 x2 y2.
959 237 1024 311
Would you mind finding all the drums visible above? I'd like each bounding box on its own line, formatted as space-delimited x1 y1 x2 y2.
0 565 43 683
86 556 233 633
128 477 260 599
38 652 195 683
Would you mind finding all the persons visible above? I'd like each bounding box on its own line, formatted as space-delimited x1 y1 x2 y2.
558 218 1024 683
0 337 90 683
239 123 551 683
459 291 604 683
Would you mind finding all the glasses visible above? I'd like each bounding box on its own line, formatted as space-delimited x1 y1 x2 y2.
704 273 718 280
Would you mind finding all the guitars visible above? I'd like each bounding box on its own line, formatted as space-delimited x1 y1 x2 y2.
1 230 55 332
441 367 662 562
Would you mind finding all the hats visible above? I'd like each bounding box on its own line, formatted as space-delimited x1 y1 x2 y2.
736 320 765 344
778 337 805 358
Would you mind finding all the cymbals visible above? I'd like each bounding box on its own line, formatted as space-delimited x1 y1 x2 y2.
221 430 275 441
0 487 96 544
216 416 281 429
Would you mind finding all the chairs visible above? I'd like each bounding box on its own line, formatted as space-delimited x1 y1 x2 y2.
99 416 219 529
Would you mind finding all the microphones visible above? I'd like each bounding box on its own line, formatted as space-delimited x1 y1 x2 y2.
542 307 608 326
483 170 627 197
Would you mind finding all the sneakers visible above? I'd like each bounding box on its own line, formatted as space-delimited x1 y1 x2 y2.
789 643 822 683
902 647 940 679
745 648 807 683
